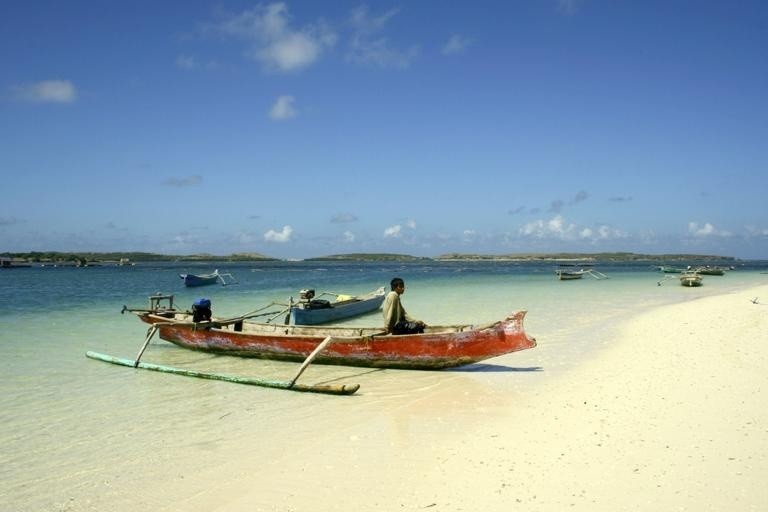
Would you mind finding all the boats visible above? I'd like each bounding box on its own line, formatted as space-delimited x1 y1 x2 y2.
177 269 217 286
1 252 134 268
658 263 728 277
681 272 703 288
291 286 386 324
132 293 538 372
556 266 587 281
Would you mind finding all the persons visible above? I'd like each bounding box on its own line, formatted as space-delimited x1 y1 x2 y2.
382 277 428 335
